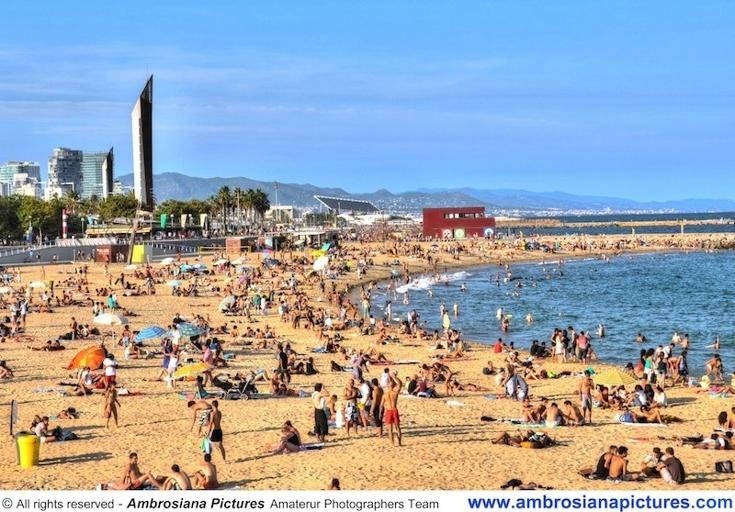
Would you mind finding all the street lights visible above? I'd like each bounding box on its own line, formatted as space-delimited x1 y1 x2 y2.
27 215 31 231
189 213 192 227
338 201 342 216
273 181 280 220
80 216 85 233
170 213 175 240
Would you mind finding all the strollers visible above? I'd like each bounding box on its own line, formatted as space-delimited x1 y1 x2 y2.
214 372 255 400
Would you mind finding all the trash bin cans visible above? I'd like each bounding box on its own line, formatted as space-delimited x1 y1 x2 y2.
15 431 40 468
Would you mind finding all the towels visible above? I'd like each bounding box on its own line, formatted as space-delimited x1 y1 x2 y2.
613 411 671 427
428 351 477 363
461 382 492 393
401 385 422 399
370 359 421 366
511 416 571 429
